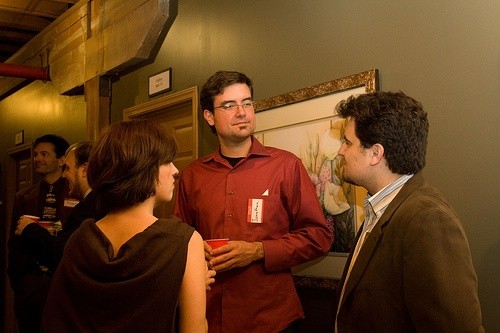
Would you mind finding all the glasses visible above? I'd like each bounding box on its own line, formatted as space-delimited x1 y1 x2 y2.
212 103 252 109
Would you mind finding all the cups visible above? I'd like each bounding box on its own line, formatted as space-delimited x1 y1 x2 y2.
39 222 51 228
24 215 39 223
206 239 230 266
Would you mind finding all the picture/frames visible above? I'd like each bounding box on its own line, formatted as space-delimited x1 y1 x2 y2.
228 68 378 291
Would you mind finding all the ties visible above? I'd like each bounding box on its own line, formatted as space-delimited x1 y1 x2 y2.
42 185 56 221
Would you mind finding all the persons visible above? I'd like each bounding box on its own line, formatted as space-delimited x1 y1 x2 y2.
172 70 333 333
41 119 216 333
8 134 107 333
334 90 487 333
